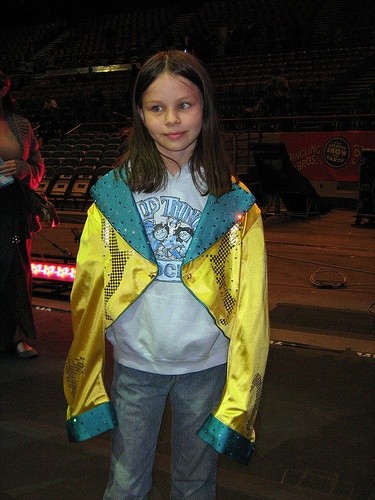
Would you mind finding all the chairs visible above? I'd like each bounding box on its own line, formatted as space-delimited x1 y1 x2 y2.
0 35 375 212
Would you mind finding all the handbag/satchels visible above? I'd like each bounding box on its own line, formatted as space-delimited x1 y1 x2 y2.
34 193 60 230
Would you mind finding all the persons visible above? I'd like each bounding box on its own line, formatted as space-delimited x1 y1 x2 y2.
64 49 270 500
0 65 44 357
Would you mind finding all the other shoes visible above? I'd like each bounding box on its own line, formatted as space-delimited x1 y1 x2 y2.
12 340 38 358
